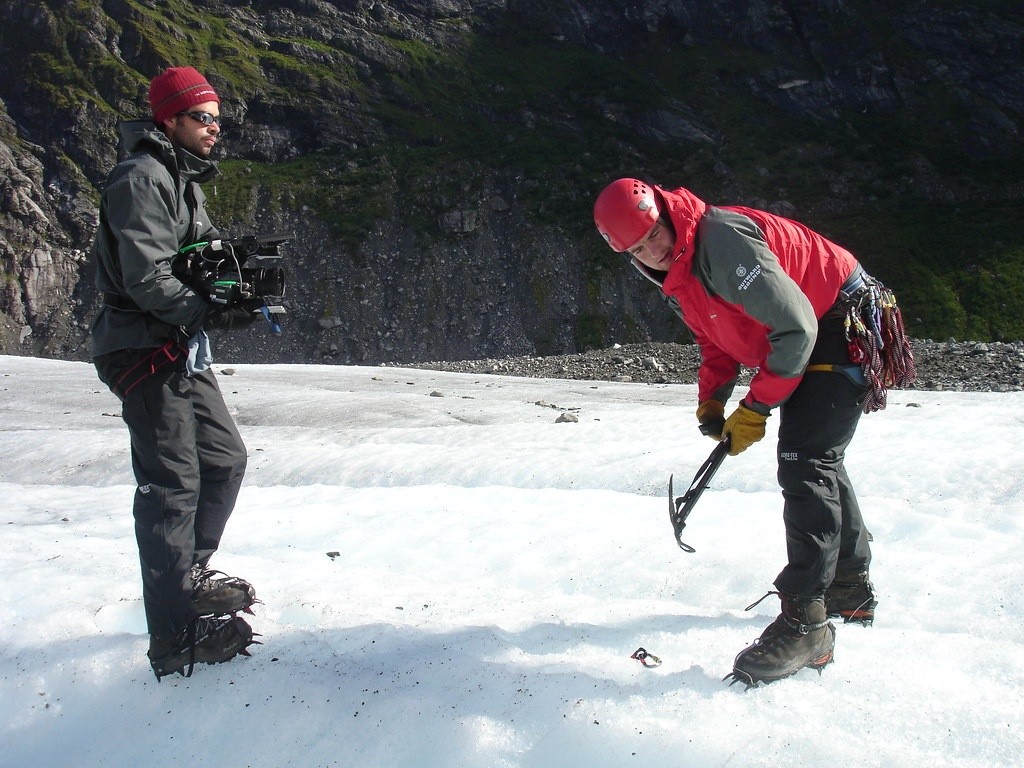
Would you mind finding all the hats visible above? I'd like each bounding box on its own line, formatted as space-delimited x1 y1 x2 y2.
149 66 220 125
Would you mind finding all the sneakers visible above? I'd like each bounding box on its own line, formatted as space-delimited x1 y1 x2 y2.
722 591 836 692
147 616 263 682
816 563 878 625
188 558 264 616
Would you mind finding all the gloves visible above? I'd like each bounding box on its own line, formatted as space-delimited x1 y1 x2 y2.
202 295 266 331
720 399 771 457
696 399 726 440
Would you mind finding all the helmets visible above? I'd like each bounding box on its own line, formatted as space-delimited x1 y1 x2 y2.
593 178 663 252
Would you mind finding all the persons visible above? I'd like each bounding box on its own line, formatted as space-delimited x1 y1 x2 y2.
90 64 261 679
595 177 894 690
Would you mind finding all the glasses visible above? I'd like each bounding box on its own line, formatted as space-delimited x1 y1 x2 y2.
176 110 223 128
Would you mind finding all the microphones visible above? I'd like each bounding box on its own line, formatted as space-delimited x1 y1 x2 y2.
240 230 297 245
